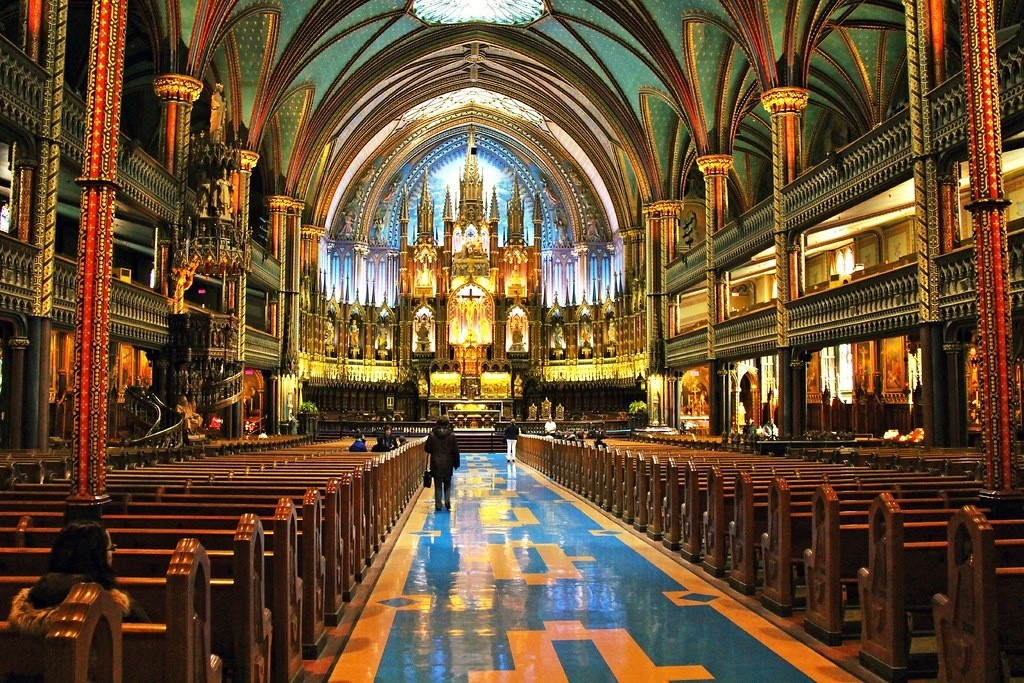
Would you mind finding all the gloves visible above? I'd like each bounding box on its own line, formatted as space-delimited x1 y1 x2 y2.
454 466 458 470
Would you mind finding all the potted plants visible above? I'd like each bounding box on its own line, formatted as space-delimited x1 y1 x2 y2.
297 401 321 435
628 400 649 429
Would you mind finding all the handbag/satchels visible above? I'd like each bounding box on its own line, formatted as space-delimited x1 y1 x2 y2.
424 470 432 488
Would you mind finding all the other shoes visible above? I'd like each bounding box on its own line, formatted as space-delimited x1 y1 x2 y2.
436 506 441 511
507 460 510 462
512 459 515 462
447 505 450 509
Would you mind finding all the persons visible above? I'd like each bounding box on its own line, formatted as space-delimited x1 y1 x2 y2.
349 428 368 452
505 419 519 462
744 419 756 434
546 429 609 448
424 416 459 509
288 413 294 421
399 436 409 446
371 436 390 452
545 416 556 435
762 418 779 439
382 425 398 449
258 429 267 438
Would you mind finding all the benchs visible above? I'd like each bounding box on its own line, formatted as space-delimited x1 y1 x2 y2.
0 436 429 683
516 431 1024 683
